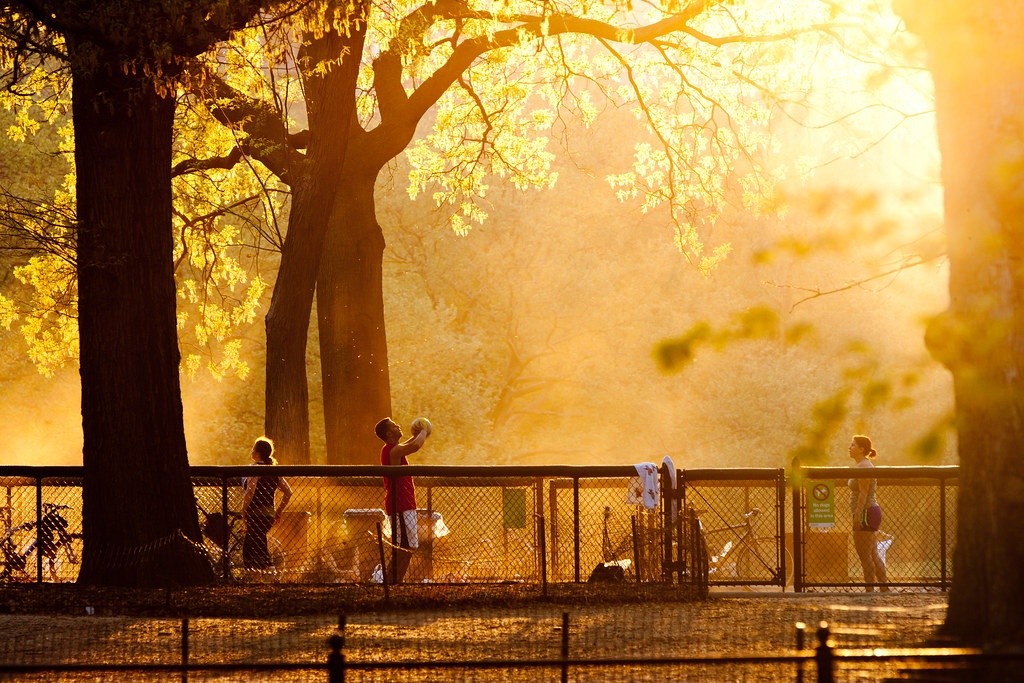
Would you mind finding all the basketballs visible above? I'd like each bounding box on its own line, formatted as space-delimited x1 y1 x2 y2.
411 417 432 437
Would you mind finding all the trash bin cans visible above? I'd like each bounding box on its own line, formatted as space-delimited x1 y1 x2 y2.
401 509 440 580
344 509 386 580
272 512 309 572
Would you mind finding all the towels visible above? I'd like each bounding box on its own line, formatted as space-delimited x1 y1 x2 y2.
662 456 678 522
626 462 658 509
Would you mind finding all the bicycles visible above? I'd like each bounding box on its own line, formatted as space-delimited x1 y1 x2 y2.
0 503 84 582
692 507 795 592
194 494 288 585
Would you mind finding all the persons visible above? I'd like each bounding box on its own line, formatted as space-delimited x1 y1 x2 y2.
373 414 428 586
239 436 293 570
848 436 891 592
